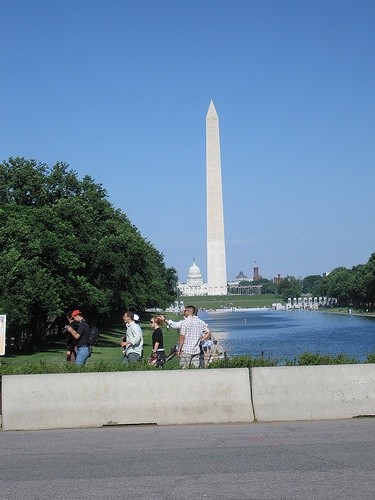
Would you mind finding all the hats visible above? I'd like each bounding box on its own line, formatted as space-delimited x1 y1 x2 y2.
71 310 82 319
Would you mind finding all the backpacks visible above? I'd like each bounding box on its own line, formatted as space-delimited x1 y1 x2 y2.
79 320 100 346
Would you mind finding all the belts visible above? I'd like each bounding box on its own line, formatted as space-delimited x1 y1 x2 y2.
77 344 89 347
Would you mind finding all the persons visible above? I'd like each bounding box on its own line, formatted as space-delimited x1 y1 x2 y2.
120 310 143 364
64 310 92 368
177 305 212 368
66 311 77 361
157 309 192 331
192 306 198 316
146 315 166 367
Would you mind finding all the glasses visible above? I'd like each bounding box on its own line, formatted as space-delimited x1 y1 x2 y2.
150 321 154 324
185 310 189 312
